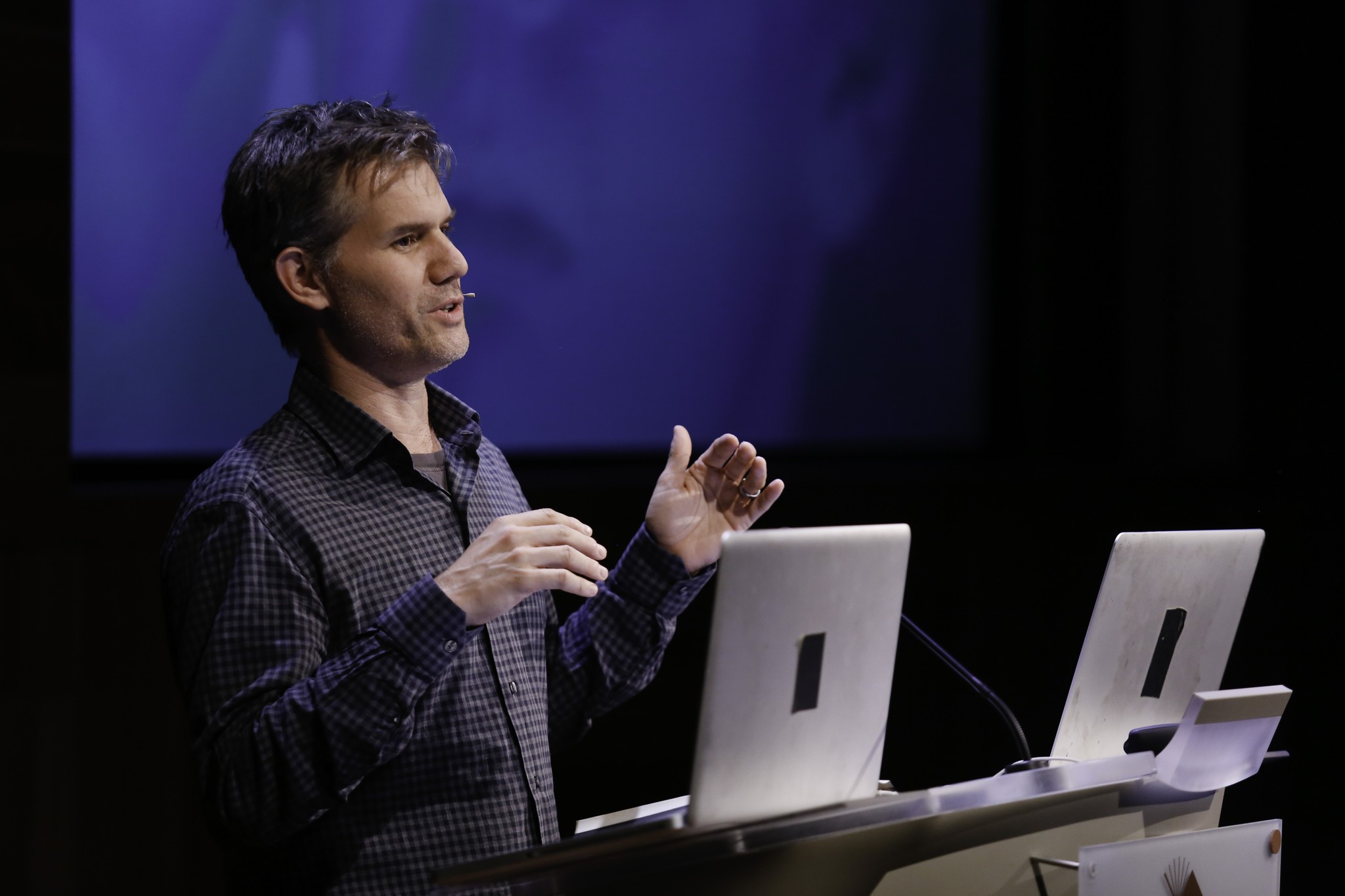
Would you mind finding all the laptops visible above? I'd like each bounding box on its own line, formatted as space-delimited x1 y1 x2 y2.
438 521 911 877
1045 528 1266 772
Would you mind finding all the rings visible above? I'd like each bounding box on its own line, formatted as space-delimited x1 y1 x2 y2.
739 478 761 498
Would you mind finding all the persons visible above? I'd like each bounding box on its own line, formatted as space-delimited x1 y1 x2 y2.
168 91 785 896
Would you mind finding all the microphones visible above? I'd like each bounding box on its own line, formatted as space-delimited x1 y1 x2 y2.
464 293 475 297
